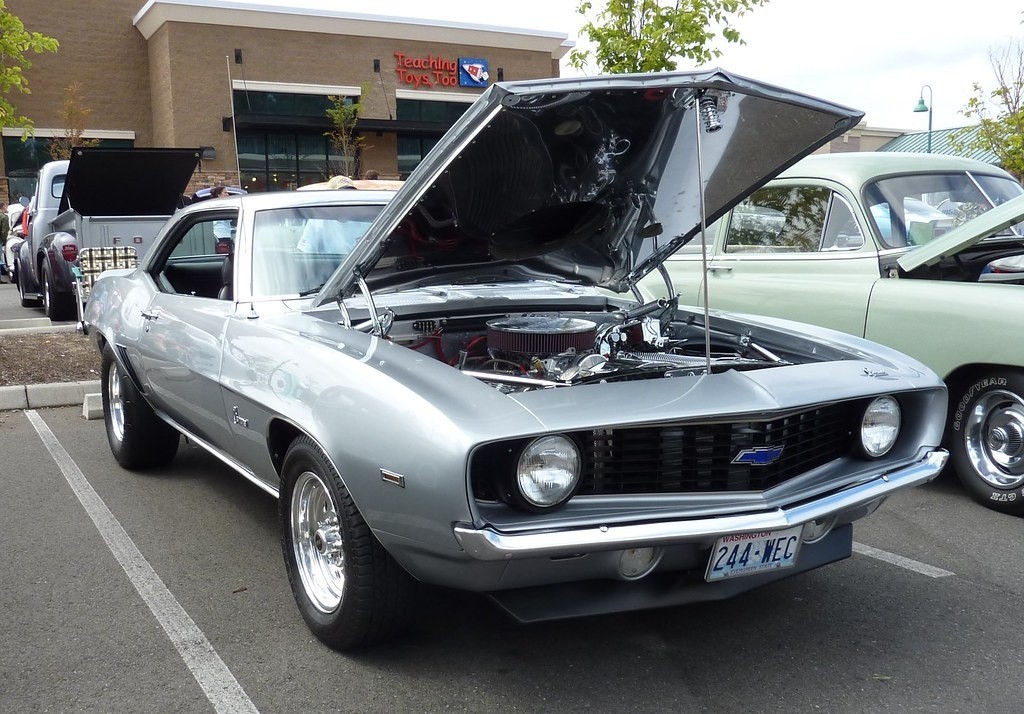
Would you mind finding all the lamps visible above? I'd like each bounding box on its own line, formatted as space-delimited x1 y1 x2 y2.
235 49 255 113
222 117 230 131
199 146 216 160
498 68 504 81
373 58 393 119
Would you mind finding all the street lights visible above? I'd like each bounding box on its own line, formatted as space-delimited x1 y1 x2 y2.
913 85 932 153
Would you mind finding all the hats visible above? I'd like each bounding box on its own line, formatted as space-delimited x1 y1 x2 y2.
327 175 357 190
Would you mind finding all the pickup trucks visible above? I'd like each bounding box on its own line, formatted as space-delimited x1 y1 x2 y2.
14 146 219 320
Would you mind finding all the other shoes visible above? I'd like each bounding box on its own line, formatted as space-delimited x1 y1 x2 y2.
0 280 7 284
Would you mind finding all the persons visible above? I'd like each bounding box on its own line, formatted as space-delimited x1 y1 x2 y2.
209 184 241 254
298 177 376 254
0 202 13 285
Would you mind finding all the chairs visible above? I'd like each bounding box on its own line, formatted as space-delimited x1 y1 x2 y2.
219 252 233 302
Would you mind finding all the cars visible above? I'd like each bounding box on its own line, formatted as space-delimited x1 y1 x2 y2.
190 180 409 253
0 203 27 283
595 152 1024 518
83 66 950 653
835 198 1009 247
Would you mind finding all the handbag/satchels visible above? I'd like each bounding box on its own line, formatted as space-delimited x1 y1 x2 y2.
215 242 230 253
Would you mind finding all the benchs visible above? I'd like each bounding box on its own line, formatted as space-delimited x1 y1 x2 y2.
166 250 350 300
